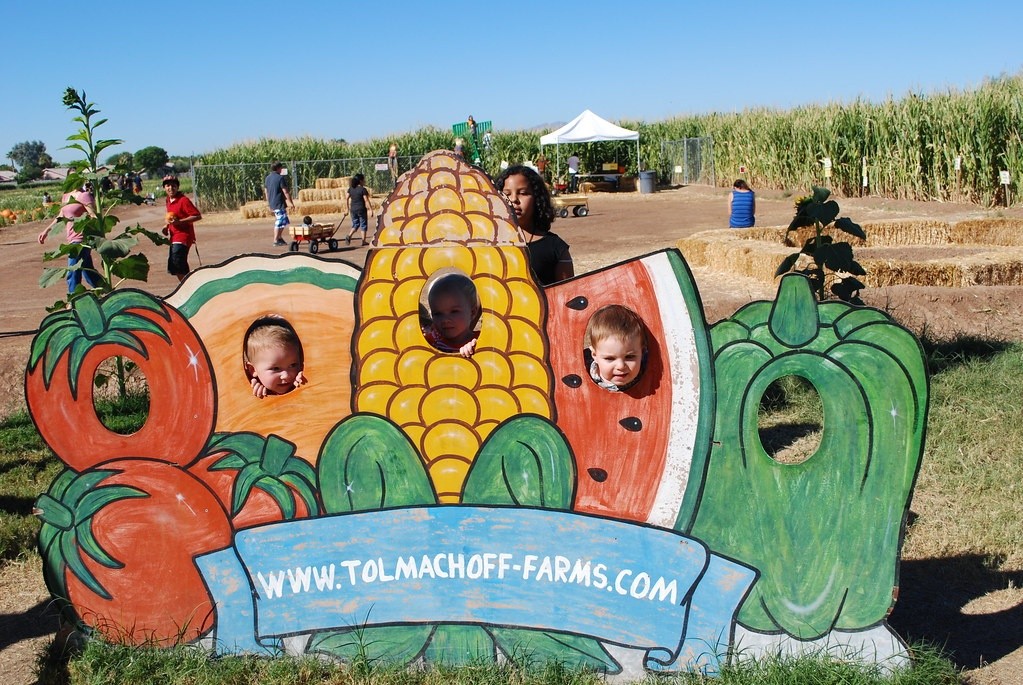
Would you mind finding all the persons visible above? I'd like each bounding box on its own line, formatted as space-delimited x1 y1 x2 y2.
727 179 756 228
493 166 574 285
162 173 202 282
301 217 325 227
344 173 374 246
603 156 618 188
468 115 477 134
419 273 481 358
482 130 492 148
584 304 647 392
38 167 99 293
42 192 51 205
83 172 142 197
244 317 303 397
567 152 582 193
264 161 295 246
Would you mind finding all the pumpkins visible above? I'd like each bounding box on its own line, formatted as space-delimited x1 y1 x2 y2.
165 212 176 221
0 208 23 220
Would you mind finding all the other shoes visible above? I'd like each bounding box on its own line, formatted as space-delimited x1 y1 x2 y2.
346 236 350 245
273 237 287 246
361 241 369 246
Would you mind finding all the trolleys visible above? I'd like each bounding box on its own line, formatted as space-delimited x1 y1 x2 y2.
288 213 346 254
551 182 596 218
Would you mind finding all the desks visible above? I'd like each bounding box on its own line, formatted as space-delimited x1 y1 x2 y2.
574 174 623 192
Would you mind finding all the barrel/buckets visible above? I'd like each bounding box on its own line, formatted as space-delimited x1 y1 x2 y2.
639 171 657 193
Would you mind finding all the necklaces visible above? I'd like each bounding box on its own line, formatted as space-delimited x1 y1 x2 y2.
526 226 534 247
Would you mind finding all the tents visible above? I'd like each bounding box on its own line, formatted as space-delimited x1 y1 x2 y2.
540 109 640 180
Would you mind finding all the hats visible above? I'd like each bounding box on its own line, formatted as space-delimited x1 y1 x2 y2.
163 175 179 187
